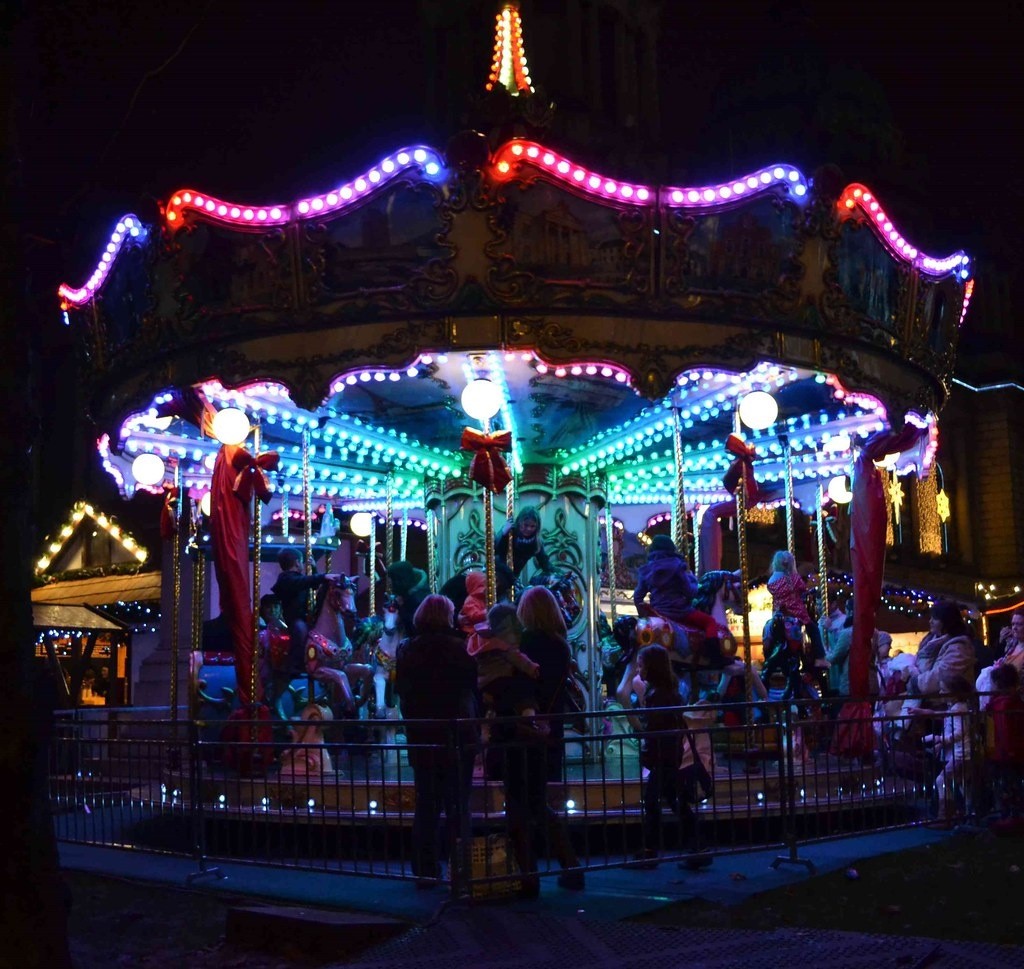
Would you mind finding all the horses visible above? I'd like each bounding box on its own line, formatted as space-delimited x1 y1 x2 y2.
375 594 402 683
613 571 765 734
598 614 627 673
257 568 371 740
759 610 801 699
513 566 590 725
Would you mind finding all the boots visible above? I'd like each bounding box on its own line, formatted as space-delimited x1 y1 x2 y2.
691 638 720 673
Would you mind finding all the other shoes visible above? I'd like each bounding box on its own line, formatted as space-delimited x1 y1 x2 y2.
517 720 550 737
451 862 475 894
413 865 442 890
952 809 965 824
925 816 954 829
558 876 584 889
630 850 658 869
679 847 713 870
516 884 540 898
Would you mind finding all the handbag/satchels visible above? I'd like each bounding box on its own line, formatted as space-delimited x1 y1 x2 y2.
673 763 714 804
448 837 528 901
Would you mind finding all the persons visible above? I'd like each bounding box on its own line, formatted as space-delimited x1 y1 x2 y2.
818 599 1024 831
396 594 483 887
80 665 110 704
762 550 831 667
456 561 585 901
632 535 736 671
386 558 434 639
494 507 555 598
630 644 715 869
680 697 716 803
257 546 348 675
